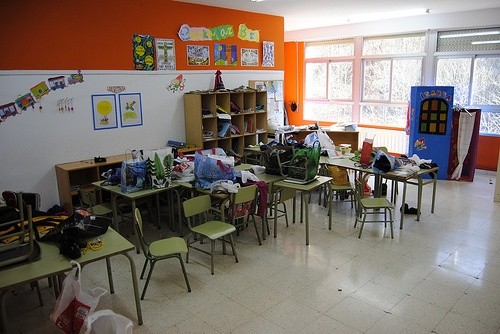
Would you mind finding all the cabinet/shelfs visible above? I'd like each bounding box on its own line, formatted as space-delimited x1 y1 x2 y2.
183 90 268 156
55 147 203 217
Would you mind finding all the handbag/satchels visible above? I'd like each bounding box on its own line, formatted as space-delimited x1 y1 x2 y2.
33 210 112 242
263 121 335 180
359 132 376 166
50 261 108 334
79 309 134 334
100 147 235 194
372 150 402 173
325 164 348 185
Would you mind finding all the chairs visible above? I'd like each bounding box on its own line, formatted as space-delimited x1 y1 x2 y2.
0 144 396 308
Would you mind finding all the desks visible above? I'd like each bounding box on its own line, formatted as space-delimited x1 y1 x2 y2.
272 174 334 247
180 162 284 256
91 179 183 255
318 150 439 230
0 210 144 326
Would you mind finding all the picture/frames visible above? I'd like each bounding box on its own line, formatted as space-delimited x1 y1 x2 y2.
118 92 143 128
91 93 119 131
